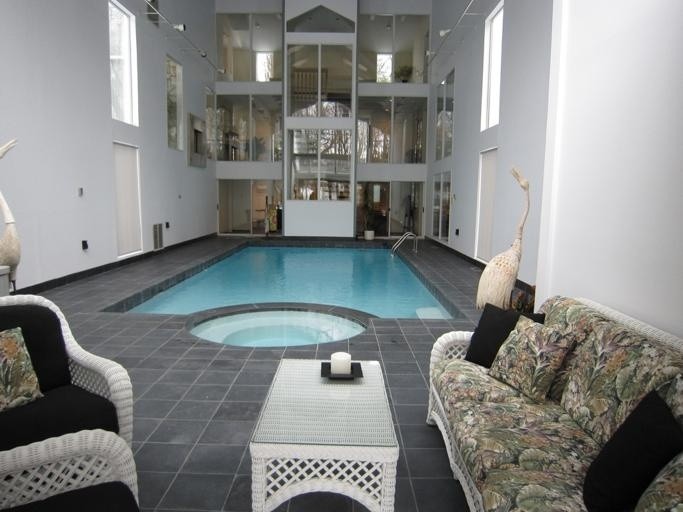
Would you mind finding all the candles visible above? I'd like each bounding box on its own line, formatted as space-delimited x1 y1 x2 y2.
328 350 352 375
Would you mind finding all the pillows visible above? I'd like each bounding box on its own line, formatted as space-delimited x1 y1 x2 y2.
0 326 43 413
583 388 682 510
464 302 575 405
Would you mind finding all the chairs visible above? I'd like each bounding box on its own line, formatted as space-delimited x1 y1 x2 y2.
0 293 140 512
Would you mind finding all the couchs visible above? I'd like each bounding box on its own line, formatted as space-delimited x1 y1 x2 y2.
426 293 683 511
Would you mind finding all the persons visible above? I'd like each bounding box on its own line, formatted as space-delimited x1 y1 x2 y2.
337 191 345 201
406 195 418 228
276 205 281 231
399 197 407 227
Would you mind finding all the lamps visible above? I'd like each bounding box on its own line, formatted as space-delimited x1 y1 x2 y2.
172 23 186 33
